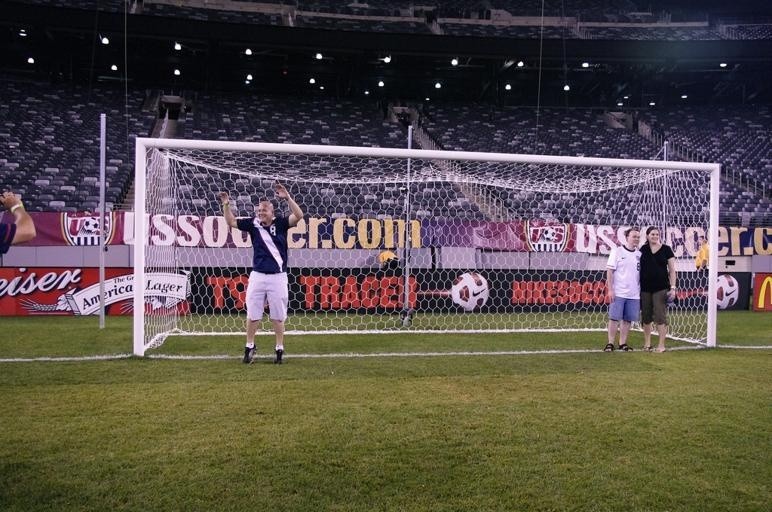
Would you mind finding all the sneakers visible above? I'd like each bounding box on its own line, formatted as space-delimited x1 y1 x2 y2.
242 343 258 366
272 346 285 364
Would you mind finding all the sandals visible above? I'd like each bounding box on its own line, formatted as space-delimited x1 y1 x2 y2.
604 343 614 352
617 342 633 353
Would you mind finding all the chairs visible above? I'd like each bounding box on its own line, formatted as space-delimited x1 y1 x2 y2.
170 89 683 161
1 80 159 212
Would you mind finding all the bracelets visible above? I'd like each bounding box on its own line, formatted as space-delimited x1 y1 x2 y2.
670 285 676 290
222 203 230 209
9 203 25 213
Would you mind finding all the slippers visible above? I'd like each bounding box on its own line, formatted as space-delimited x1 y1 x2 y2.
641 345 666 354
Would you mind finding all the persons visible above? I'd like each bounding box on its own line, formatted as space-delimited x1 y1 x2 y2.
693 235 709 271
0 191 39 267
602 228 643 353
640 226 677 354
217 184 303 367
399 108 409 122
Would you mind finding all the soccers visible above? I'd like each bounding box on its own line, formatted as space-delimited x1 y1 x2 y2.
715 274 739 309
450 273 490 313
